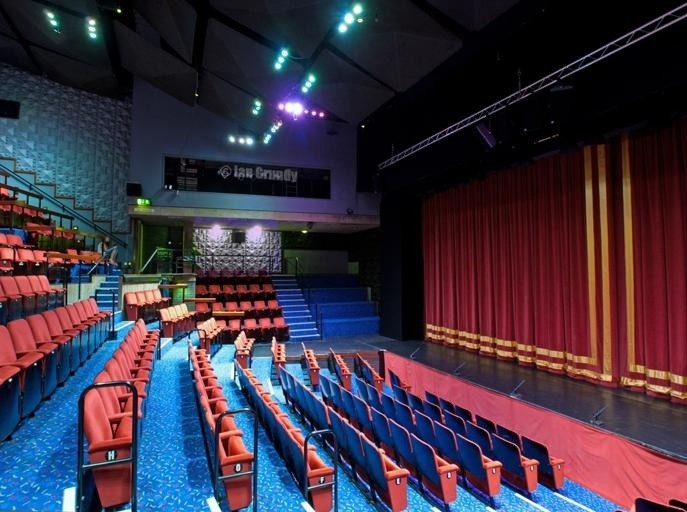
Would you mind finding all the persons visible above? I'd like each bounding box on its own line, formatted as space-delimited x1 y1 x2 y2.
98 234 120 267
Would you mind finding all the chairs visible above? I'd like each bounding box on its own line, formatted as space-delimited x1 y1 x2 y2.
0 184 157 512
124 269 286 356
186 343 562 511
